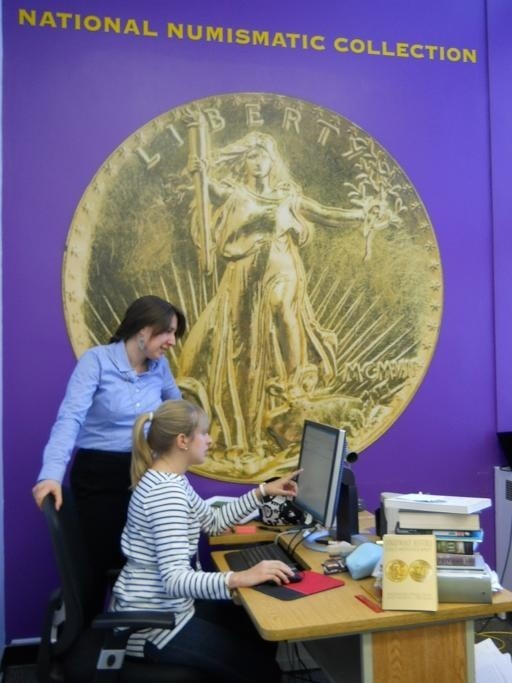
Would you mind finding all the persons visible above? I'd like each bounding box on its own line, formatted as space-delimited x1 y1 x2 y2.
103 397 307 682
28 295 186 560
174 131 402 465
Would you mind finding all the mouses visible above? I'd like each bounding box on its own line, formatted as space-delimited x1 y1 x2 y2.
265 572 304 586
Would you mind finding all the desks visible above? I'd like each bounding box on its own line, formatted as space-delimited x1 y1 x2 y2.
205 508 512 681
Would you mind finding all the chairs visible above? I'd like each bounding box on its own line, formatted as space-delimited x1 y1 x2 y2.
34 492 176 681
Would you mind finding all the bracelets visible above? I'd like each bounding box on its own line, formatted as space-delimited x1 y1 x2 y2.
258 481 266 498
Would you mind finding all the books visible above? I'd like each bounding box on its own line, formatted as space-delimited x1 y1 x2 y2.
383 491 492 571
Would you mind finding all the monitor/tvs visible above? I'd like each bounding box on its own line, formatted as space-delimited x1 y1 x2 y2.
290 419 358 552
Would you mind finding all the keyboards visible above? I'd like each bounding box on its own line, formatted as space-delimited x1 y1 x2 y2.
224 544 298 573
